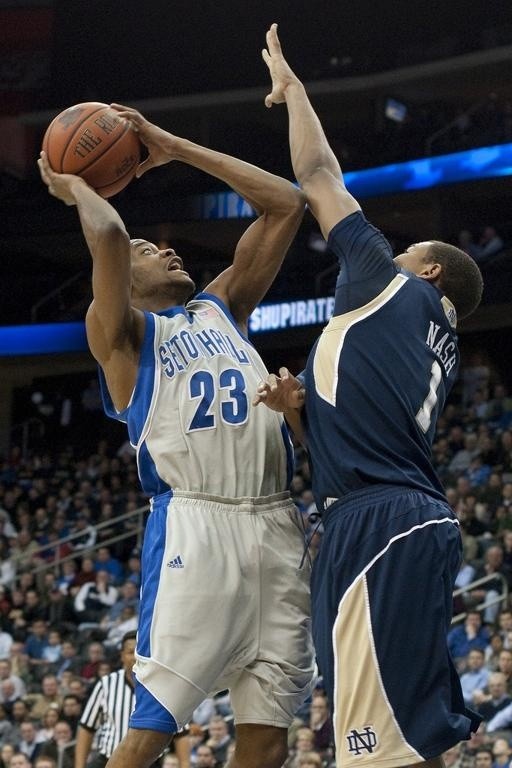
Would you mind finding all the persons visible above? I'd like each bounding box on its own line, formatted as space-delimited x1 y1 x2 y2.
33 100 319 768
251 21 484 766
75 630 191 768
1 222 511 768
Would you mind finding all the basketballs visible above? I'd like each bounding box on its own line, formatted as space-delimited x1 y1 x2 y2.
43 102 141 198
